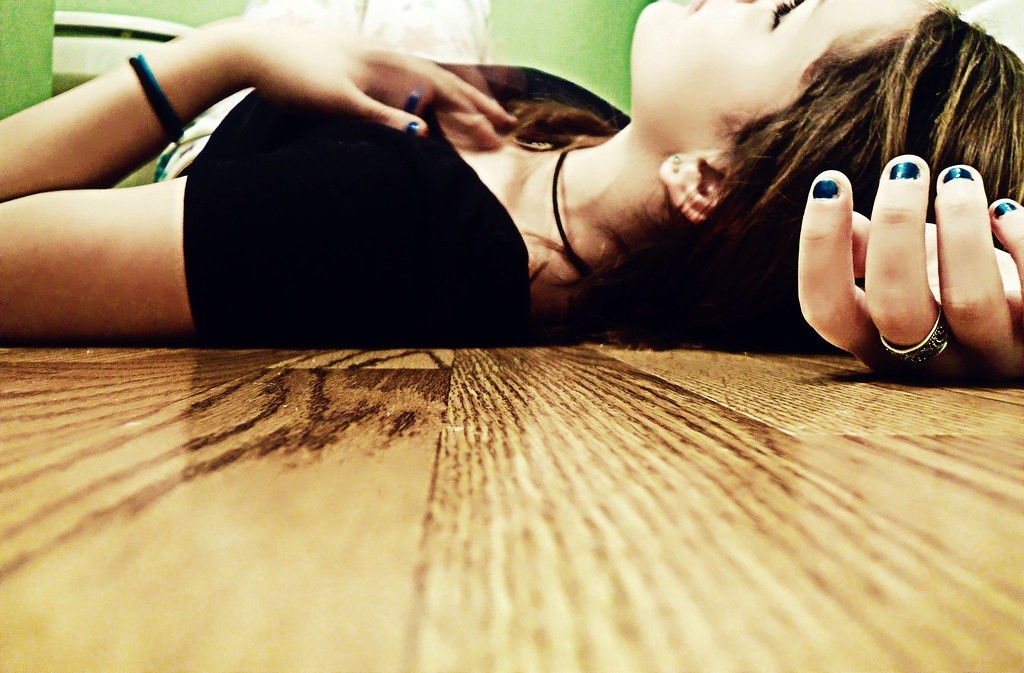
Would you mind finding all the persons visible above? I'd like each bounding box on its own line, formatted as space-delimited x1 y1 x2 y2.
0 2 1024 387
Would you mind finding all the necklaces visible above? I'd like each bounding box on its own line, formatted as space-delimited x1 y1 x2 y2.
553 147 596 275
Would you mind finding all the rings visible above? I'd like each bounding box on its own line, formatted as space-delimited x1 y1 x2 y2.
880 305 951 366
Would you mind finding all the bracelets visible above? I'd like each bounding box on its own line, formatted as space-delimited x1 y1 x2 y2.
127 53 180 141
